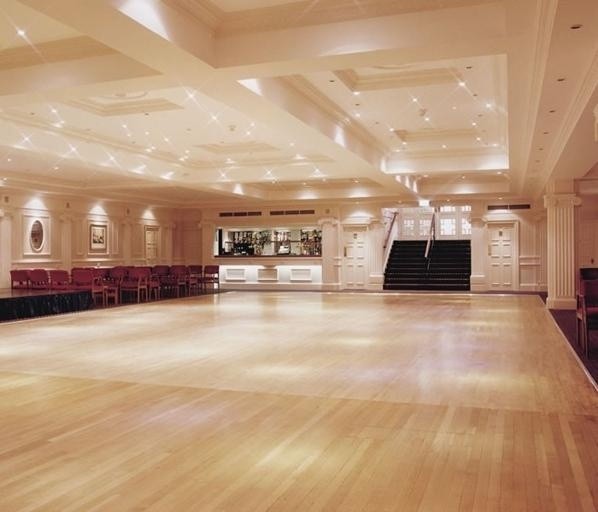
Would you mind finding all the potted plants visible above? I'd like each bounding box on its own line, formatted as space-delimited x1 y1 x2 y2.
90 224 107 251
29 219 46 251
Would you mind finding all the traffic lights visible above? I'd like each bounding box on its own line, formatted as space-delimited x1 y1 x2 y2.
579 280 598 307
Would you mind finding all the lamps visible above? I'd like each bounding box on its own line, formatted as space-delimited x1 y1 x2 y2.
576 268 598 357
10 265 219 308
